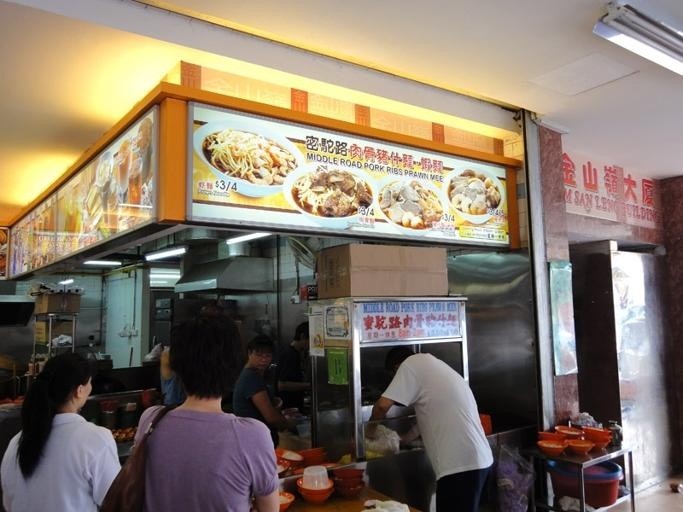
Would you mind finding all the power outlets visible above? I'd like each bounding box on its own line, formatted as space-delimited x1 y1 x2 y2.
290 296 300 304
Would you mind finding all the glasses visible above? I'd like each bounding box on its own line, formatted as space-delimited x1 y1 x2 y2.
253 353 271 363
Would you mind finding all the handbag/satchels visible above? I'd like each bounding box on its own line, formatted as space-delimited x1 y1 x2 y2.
99 401 184 512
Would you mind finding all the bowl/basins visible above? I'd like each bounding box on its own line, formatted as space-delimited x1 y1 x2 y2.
583 426 612 449
555 425 585 439
280 492 296 511
539 432 567 440
334 468 366 497
295 467 331 502
568 439 596 456
276 444 331 468
548 461 624 507
537 439 568 457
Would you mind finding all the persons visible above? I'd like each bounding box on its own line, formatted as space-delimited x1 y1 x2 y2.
160 345 187 405
367 346 494 512
232 337 279 425
99 334 279 512
281 322 311 369
0 351 121 511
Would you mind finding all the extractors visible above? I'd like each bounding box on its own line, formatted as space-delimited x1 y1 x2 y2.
175 244 275 291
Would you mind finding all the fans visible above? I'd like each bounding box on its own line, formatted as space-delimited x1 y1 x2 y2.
287 236 320 270
